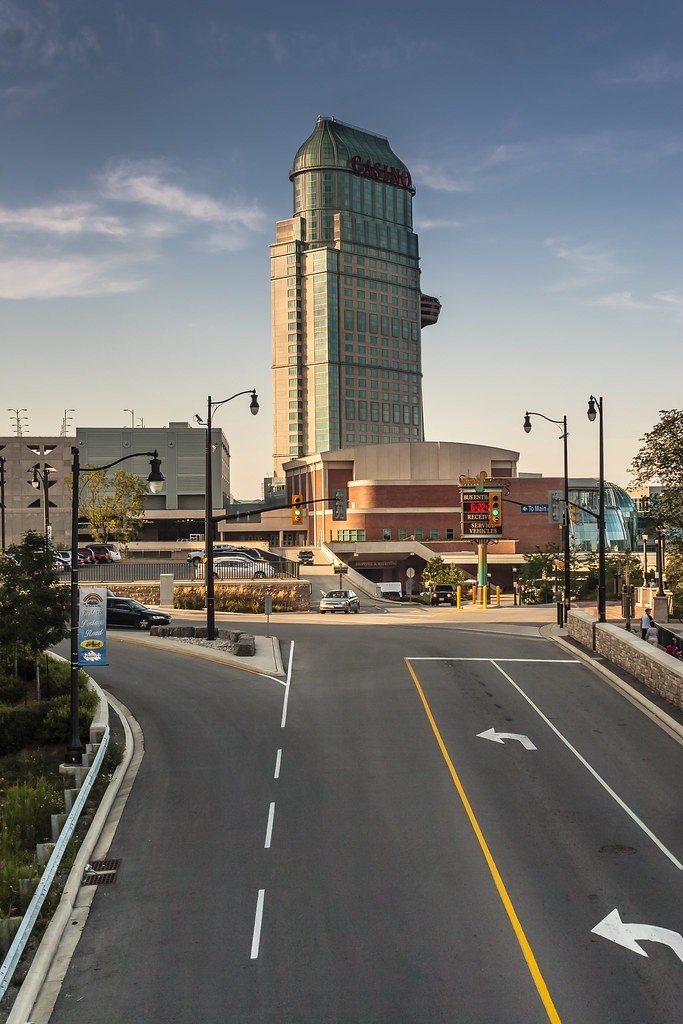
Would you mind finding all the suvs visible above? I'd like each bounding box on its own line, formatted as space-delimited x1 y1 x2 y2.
431 584 457 607
186 544 270 565
83 543 121 562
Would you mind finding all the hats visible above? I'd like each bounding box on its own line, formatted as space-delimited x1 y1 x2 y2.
645 606 652 612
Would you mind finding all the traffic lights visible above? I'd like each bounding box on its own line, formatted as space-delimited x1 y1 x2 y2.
488 492 501 527
569 496 576 524
291 495 303 525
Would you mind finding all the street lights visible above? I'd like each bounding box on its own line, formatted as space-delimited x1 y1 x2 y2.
124 409 136 428
656 524 669 597
64 445 166 765
523 410 571 624
586 395 605 623
31 467 51 554
641 530 649 587
62 409 75 437
8 408 29 437
192 388 260 642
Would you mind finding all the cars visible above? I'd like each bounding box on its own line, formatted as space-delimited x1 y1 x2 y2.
77 597 172 630
319 589 360 614
297 550 314 565
463 585 474 600
0 547 95 575
56 588 115 605
196 556 275 580
91 546 112 564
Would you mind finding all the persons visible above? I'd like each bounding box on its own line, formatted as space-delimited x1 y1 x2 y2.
666 638 682 658
639 608 660 640
646 621 659 648
647 578 655 588
